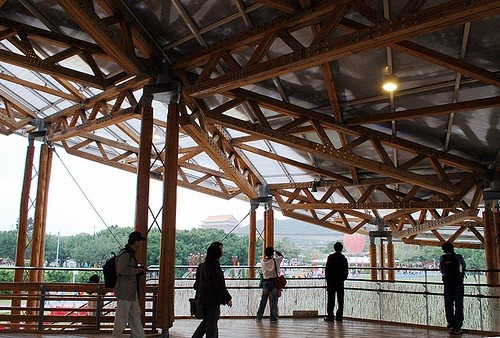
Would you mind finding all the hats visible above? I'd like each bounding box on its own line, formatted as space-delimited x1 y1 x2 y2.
128 231 147 241
87 274 99 283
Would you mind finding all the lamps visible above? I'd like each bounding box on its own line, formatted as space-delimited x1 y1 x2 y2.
381 63 398 92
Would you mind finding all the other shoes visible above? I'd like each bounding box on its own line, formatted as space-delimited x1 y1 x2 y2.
255 317 261 321
449 328 454 334
270 319 278 323
335 316 342 322
324 316 334 322
454 328 463 334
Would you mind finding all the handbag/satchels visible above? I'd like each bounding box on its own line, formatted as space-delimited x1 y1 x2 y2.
188 297 203 319
278 275 287 289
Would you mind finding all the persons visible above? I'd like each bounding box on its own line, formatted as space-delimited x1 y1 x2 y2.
192 241 232 338
256 247 283 323
109 231 145 338
440 242 466 329
324 242 348 321
88 274 103 316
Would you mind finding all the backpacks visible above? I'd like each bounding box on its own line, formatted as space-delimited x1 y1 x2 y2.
102 248 131 289
440 254 467 285
